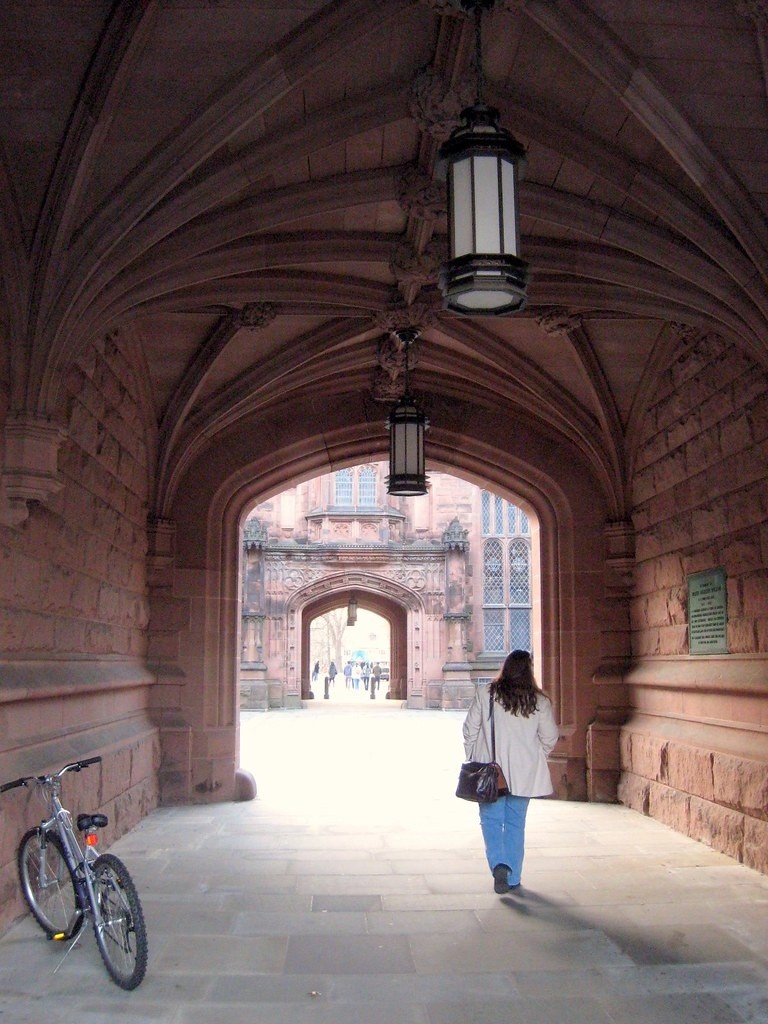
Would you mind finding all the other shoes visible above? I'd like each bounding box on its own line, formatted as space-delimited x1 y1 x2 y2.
509 883 520 890
493 864 509 894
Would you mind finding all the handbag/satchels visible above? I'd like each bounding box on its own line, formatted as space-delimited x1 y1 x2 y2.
455 762 509 803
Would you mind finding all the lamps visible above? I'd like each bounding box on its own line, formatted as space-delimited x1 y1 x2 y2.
432 0 529 317
347 591 358 626
382 328 432 497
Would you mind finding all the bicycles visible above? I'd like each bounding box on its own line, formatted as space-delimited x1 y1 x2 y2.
0 756 148 992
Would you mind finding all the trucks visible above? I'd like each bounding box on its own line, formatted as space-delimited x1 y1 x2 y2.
374 661 389 680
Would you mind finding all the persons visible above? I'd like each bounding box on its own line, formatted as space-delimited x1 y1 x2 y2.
344 661 373 690
314 661 319 682
372 662 381 690
328 662 337 687
462 650 559 894
